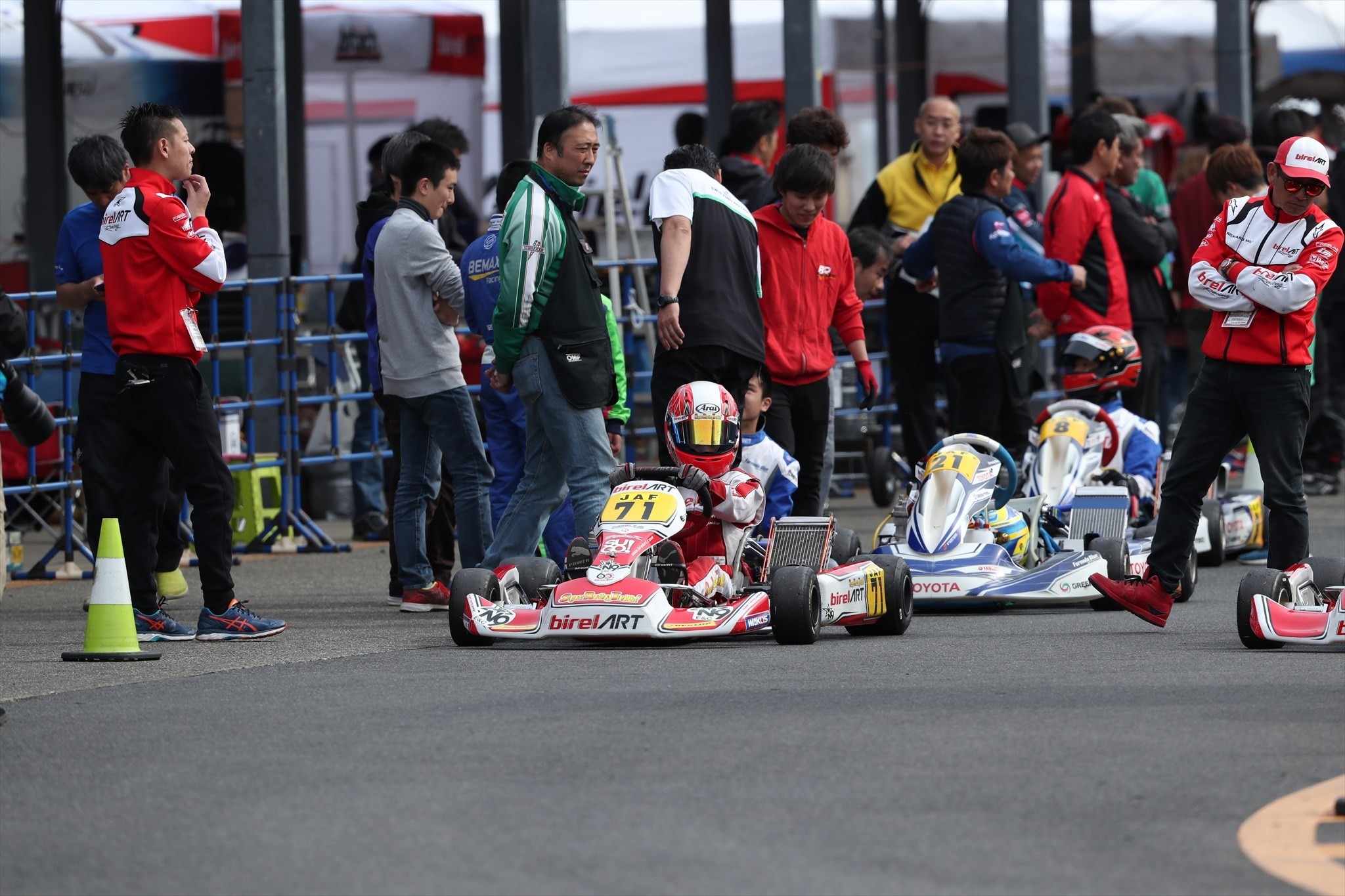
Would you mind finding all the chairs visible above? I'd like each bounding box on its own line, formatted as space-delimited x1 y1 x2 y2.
0 398 87 545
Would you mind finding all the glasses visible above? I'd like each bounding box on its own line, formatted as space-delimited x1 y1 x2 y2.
1276 167 1326 196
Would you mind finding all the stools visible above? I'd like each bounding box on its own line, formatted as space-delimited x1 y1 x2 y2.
224 452 294 543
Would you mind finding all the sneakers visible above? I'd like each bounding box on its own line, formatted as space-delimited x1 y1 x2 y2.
1088 566 1182 628
400 580 451 613
132 596 197 642
195 598 288 642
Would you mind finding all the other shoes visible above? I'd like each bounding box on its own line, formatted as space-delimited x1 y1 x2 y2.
156 567 188 600
564 537 593 582
352 517 390 541
656 540 686 608
387 587 403 605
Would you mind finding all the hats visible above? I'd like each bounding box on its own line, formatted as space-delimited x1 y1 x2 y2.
1006 119 1051 149
1273 136 1331 188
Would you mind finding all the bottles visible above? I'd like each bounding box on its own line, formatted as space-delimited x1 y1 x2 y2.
219 409 241 456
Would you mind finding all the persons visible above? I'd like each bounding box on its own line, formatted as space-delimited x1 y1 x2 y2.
649 98 1345 539
338 102 632 610
55 134 189 609
564 380 765 608
99 103 287 643
1089 137 1345 627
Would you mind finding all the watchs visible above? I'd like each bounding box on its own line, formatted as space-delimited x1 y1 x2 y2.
656 295 679 308
1220 257 1240 279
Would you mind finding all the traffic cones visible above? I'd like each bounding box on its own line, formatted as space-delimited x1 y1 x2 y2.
60 516 163 661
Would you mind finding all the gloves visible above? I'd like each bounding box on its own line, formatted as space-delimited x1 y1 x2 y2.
854 360 879 411
608 462 636 488
678 464 711 491
1093 469 1139 497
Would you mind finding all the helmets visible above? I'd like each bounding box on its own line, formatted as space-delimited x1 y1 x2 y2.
664 380 741 478
1062 324 1143 393
987 504 1031 566
